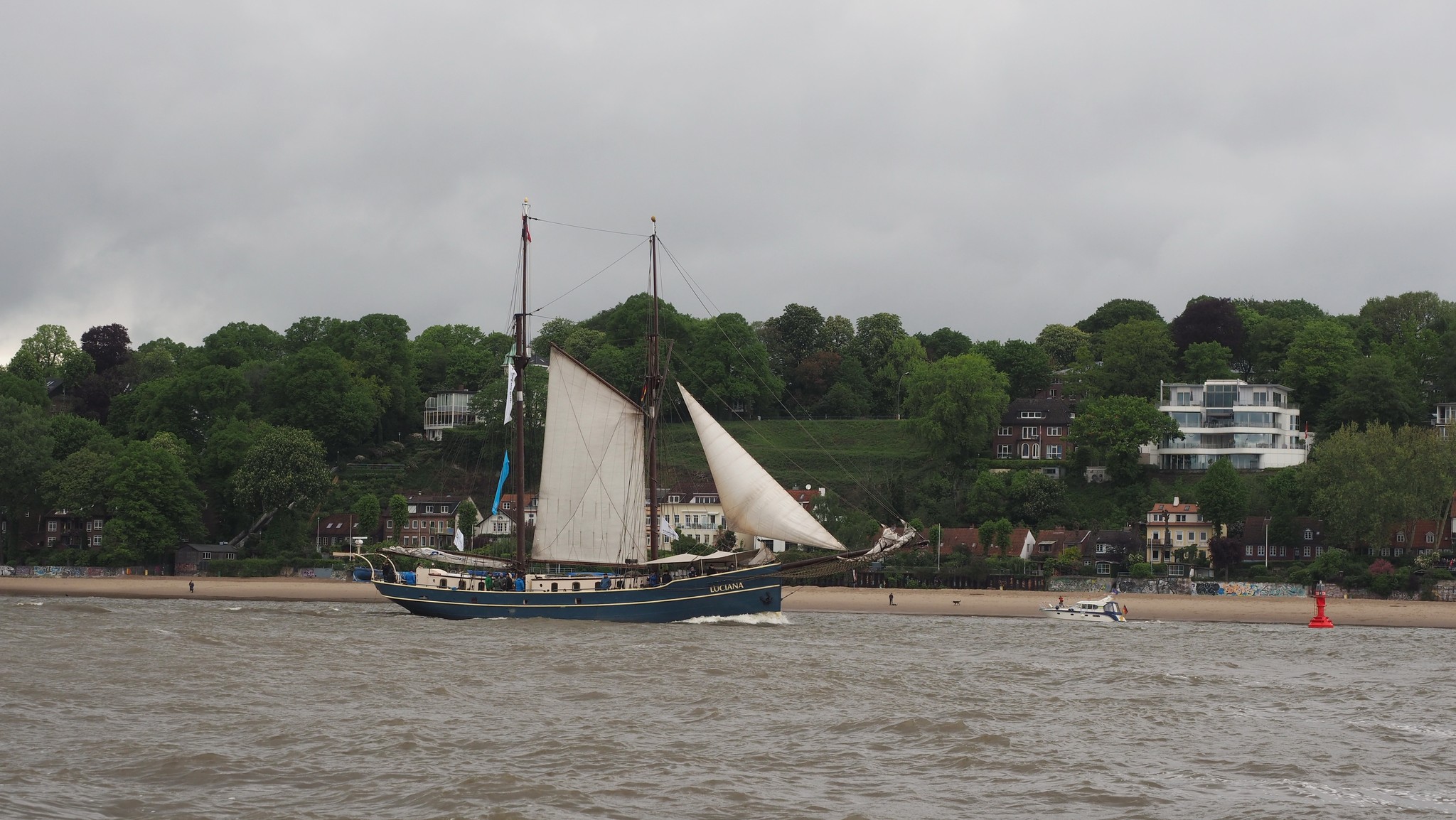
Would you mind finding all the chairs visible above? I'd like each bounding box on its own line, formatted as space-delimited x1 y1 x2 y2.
689 523 700 529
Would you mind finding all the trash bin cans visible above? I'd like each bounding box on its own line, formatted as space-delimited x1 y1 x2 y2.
872 562 881 571
879 584 883 588
1000 586 1003 590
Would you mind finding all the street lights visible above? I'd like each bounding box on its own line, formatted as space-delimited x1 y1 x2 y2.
317 517 320 553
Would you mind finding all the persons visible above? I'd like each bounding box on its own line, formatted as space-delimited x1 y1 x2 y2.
405 569 416 587
600 573 612 590
707 564 716 575
889 593 893 605
387 568 396 583
485 572 513 592
662 570 672 586
514 572 542 593
189 580 194 593
1088 595 1090 601
646 570 657 588
1450 560 1453 567
689 567 697 578
728 563 735 572
1059 596 1063 603
382 562 391 582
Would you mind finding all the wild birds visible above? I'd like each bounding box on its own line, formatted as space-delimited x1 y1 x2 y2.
65 594 69 596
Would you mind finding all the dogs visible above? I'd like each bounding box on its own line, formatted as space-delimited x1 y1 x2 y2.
953 600 961 606
892 603 898 606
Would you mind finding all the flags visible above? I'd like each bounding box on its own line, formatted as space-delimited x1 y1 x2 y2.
1123 604 1128 614
492 450 510 516
504 363 517 425
1305 421 1308 439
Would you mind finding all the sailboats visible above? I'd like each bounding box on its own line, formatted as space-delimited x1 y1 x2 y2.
332 196 931 624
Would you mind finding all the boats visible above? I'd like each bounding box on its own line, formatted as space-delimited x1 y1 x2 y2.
1038 587 1126 622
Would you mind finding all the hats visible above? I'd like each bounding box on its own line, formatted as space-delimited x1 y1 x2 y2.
710 564 713 566
664 570 669 572
651 570 655 572
603 573 607 575
691 566 694 569
488 571 492 574
891 593 892 594
500 573 503 576
730 563 736 566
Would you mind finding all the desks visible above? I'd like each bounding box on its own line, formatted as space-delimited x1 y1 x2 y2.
705 524 711 529
